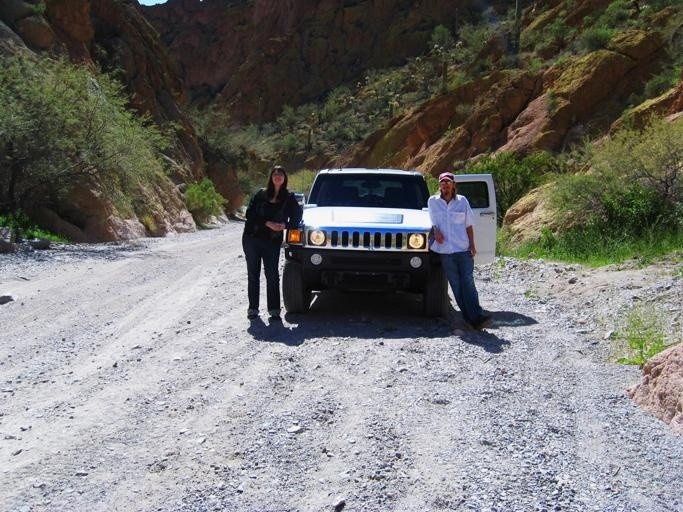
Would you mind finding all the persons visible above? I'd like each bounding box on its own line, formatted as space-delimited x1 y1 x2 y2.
424 171 492 332
239 164 303 321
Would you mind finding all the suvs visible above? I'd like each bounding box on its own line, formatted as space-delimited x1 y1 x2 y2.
281 166 498 314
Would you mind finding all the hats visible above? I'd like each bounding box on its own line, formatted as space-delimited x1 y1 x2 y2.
438 172 454 182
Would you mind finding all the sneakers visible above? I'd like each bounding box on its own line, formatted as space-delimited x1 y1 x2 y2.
247 308 258 318
271 313 281 321
471 316 491 328
